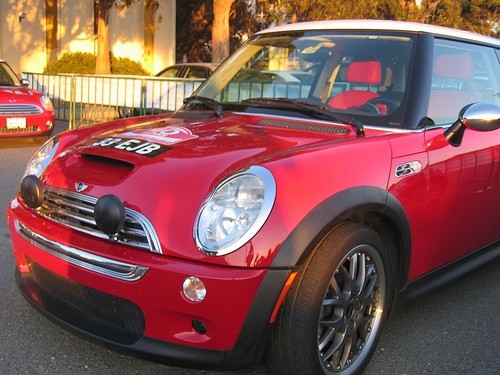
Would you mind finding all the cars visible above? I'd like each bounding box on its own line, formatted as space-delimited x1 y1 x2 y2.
258 68 314 99
0 59 55 138
118 61 279 119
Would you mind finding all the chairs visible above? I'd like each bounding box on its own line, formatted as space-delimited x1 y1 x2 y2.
426 53 480 118
329 60 388 114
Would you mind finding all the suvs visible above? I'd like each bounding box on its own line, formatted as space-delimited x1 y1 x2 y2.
5 16 500 375
303 60 379 98
